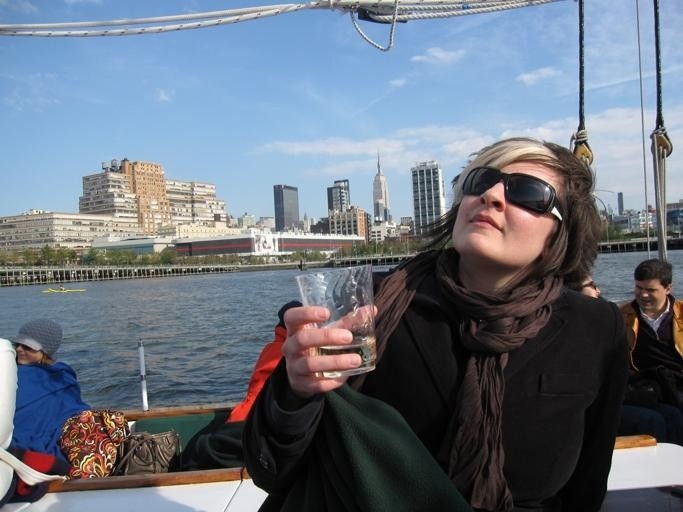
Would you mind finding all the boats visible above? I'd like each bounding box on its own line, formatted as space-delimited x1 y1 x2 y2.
48 287 85 293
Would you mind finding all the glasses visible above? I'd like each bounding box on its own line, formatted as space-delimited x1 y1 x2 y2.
461 166 563 222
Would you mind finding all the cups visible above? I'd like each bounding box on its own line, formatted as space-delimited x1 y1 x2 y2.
295 263 378 379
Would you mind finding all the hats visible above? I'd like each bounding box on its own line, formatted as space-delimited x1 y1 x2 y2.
10 319 63 355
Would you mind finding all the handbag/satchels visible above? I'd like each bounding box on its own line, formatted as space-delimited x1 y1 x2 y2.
57 409 131 478
109 430 182 475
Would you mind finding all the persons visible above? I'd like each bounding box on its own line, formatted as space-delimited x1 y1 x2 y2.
11 314 64 364
239 135 682 510
576 272 682 444
616 257 683 375
223 300 304 423
